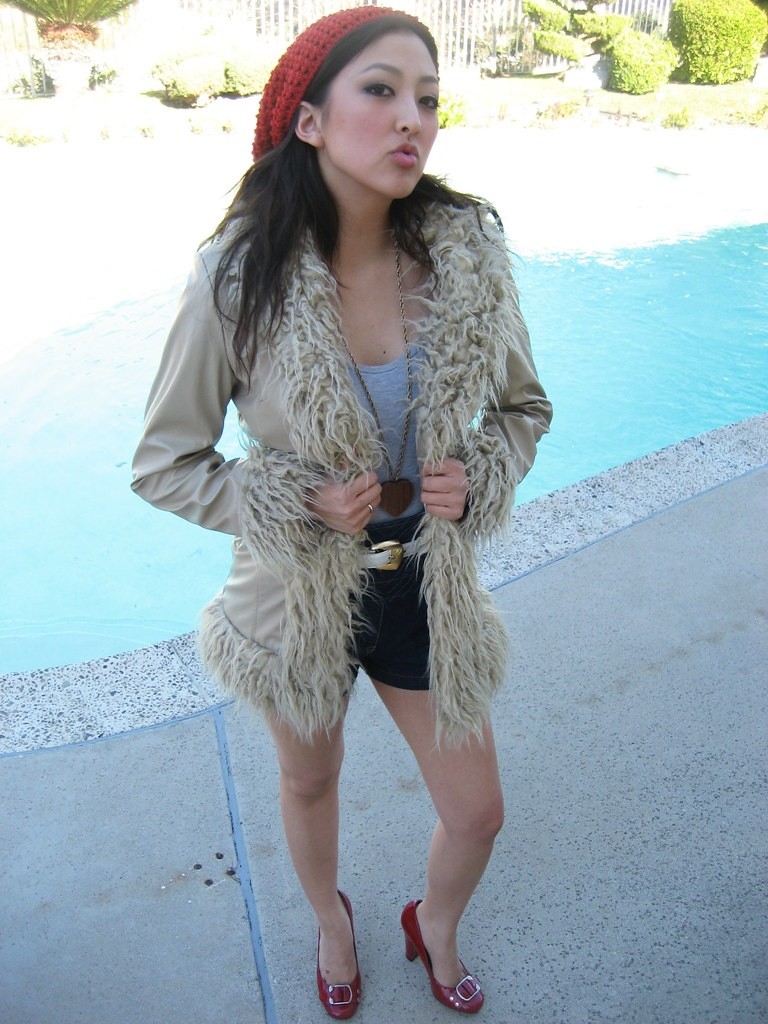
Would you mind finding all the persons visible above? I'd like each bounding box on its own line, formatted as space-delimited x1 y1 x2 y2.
130 3 553 1021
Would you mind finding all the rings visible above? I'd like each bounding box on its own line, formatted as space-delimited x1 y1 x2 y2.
367 503 374 513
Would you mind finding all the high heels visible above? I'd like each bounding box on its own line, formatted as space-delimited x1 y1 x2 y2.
401 899 484 1014
317 889 363 1021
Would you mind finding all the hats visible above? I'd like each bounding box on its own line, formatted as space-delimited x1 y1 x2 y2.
251 7 438 162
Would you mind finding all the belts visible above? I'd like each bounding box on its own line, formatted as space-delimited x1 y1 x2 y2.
359 539 421 570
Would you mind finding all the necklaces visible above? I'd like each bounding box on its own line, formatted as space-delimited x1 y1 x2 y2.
336 229 414 518
